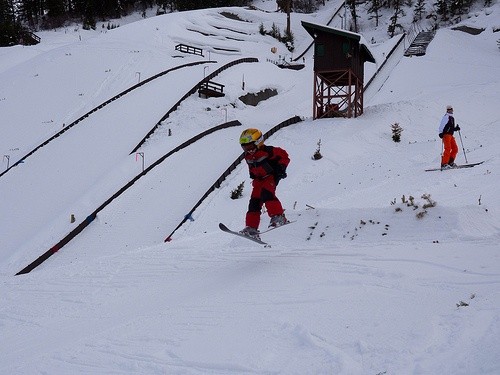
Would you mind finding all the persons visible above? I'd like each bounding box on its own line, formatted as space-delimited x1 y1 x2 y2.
238 129 290 235
438 106 460 170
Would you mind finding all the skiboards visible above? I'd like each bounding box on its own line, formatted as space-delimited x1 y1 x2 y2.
219 220 296 249
424 160 485 172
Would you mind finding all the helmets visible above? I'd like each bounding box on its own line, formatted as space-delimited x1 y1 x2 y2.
447 105 452 110
239 128 264 149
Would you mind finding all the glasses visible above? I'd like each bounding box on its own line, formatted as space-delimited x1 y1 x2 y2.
242 142 256 150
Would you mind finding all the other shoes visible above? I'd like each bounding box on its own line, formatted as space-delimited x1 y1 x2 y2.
448 162 454 166
441 164 448 168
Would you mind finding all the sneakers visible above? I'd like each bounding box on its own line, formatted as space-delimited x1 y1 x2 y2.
268 214 285 228
240 226 259 239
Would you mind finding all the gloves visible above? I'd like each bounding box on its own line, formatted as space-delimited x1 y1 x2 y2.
272 164 288 185
456 126 460 130
439 133 443 138
